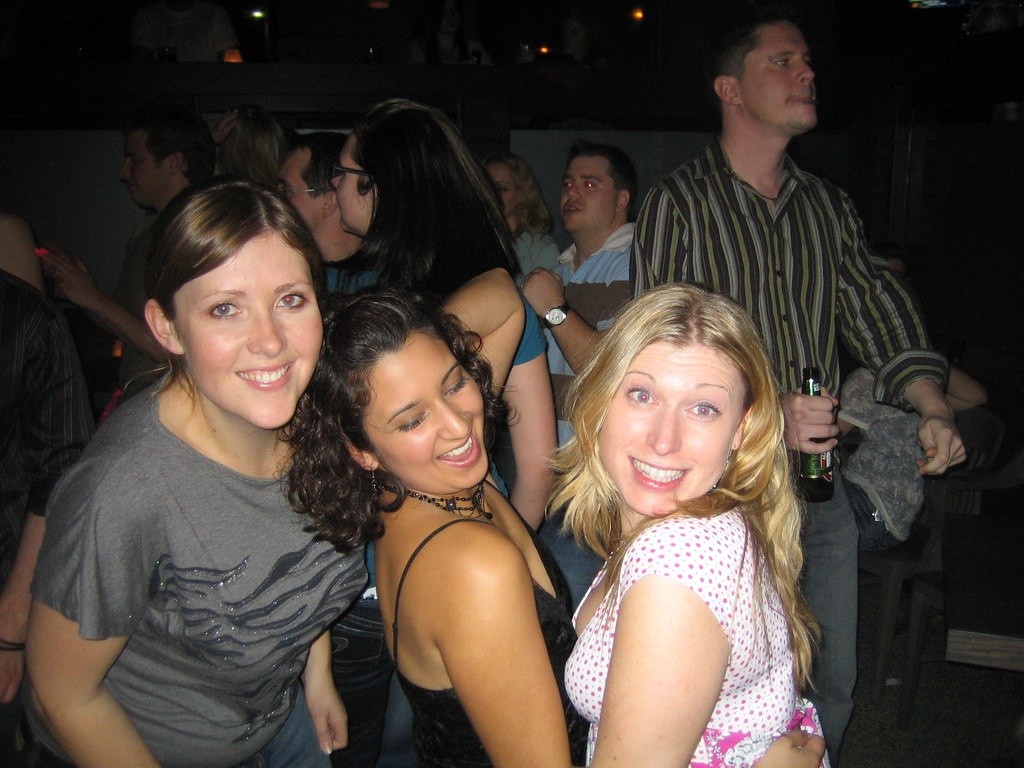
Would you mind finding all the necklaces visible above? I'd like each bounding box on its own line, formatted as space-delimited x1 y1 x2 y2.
380 481 493 521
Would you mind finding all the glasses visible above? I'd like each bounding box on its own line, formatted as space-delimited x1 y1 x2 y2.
332 162 369 177
276 182 336 199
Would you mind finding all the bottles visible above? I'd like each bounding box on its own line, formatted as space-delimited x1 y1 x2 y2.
796 367 834 503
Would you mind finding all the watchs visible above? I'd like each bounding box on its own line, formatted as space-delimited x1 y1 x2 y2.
543 303 572 329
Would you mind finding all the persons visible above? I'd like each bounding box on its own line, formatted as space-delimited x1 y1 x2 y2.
628 8 990 768
24 175 371 768
281 287 592 768
543 281 833 768
0 0 1024 768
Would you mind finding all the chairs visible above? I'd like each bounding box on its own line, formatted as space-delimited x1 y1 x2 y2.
855 408 1024 731
